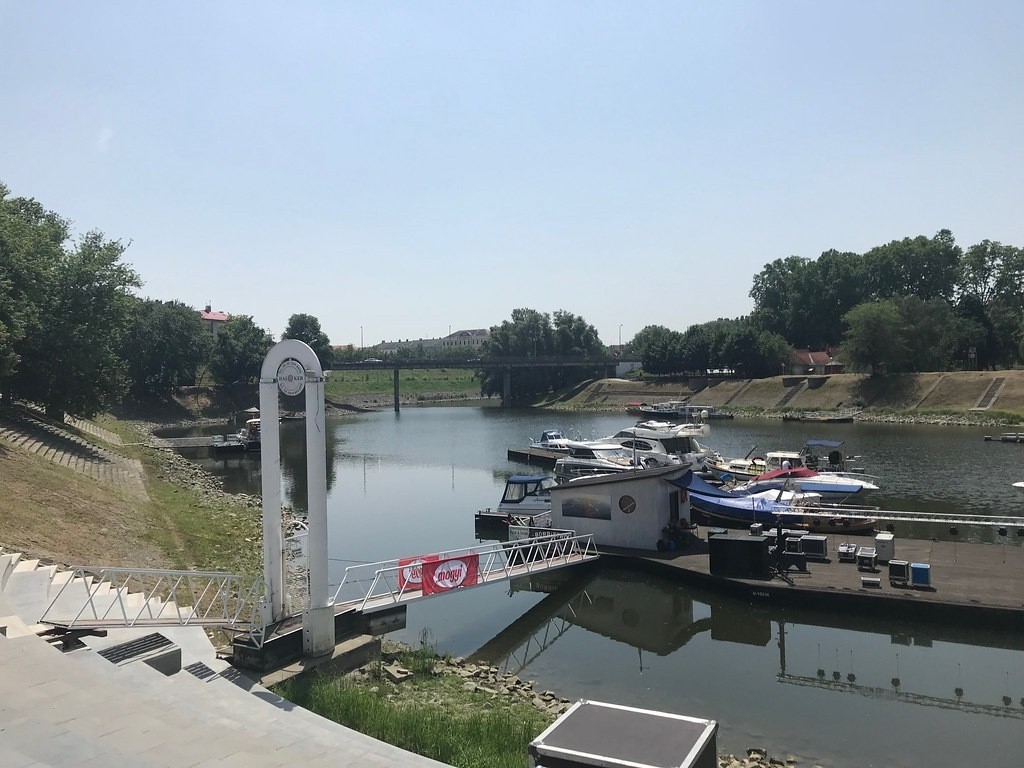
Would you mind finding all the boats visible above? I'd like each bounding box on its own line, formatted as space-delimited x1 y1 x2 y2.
528 429 570 454
639 393 734 419
691 438 880 535
621 402 648 413
496 408 725 514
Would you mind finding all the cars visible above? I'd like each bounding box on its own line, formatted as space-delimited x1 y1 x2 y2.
365 358 383 363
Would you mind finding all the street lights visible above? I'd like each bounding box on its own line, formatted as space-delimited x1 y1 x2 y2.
448 325 452 367
361 326 363 364
619 324 624 359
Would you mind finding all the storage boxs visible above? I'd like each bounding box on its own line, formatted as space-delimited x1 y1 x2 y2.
888 560 909 586
837 543 856 559
862 577 884 588
875 534 895 561
910 563 931 588
527 698 720 768
748 522 827 559
856 546 878 572
